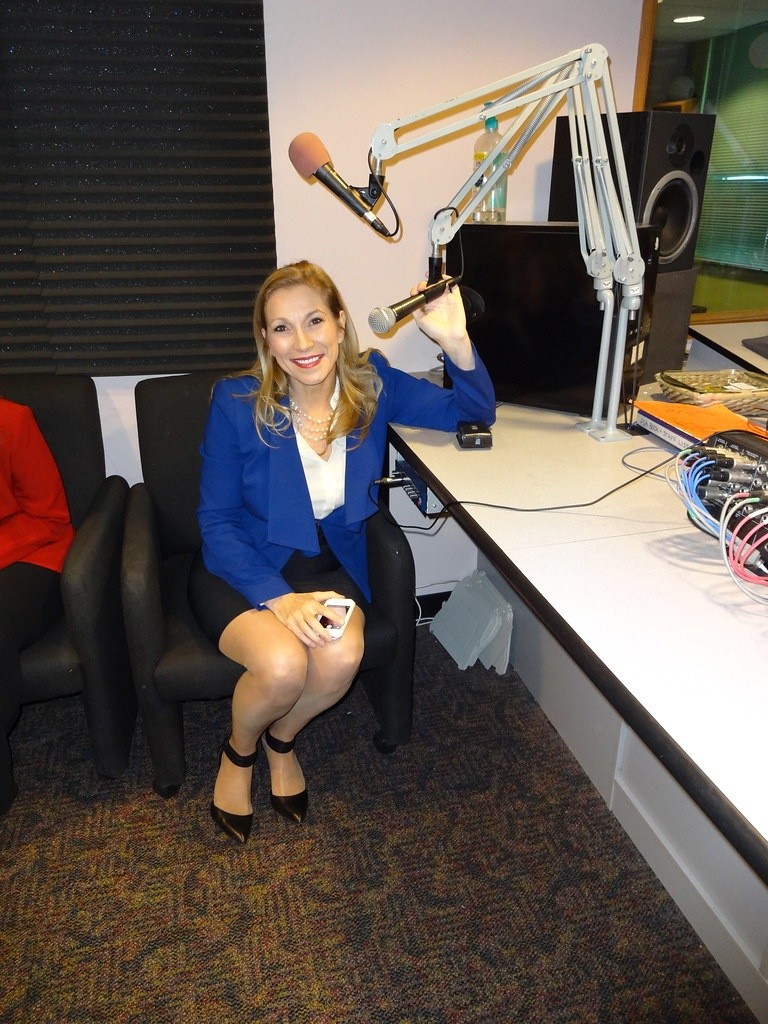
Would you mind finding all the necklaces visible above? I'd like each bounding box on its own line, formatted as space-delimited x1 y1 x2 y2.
289 401 332 442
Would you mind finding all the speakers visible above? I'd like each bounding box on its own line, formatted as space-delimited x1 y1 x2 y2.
548 110 717 273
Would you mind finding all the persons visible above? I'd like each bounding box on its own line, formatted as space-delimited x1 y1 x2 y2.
0 399 76 816
187 259 496 845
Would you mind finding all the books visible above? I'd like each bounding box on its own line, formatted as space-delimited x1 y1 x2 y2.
636 412 700 450
633 400 768 444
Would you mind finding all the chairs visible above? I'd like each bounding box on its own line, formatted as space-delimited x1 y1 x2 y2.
0 372 129 779
123 370 416 798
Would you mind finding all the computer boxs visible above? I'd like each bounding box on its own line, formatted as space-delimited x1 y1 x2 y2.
440 221 663 420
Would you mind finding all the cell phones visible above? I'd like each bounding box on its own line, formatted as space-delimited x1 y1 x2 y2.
317 598 355 640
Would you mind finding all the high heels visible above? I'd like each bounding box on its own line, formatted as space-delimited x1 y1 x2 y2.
211 736 259 844
260 729 308 824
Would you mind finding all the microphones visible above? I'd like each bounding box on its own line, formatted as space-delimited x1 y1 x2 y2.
288 132 390 238
368 275 463 334
